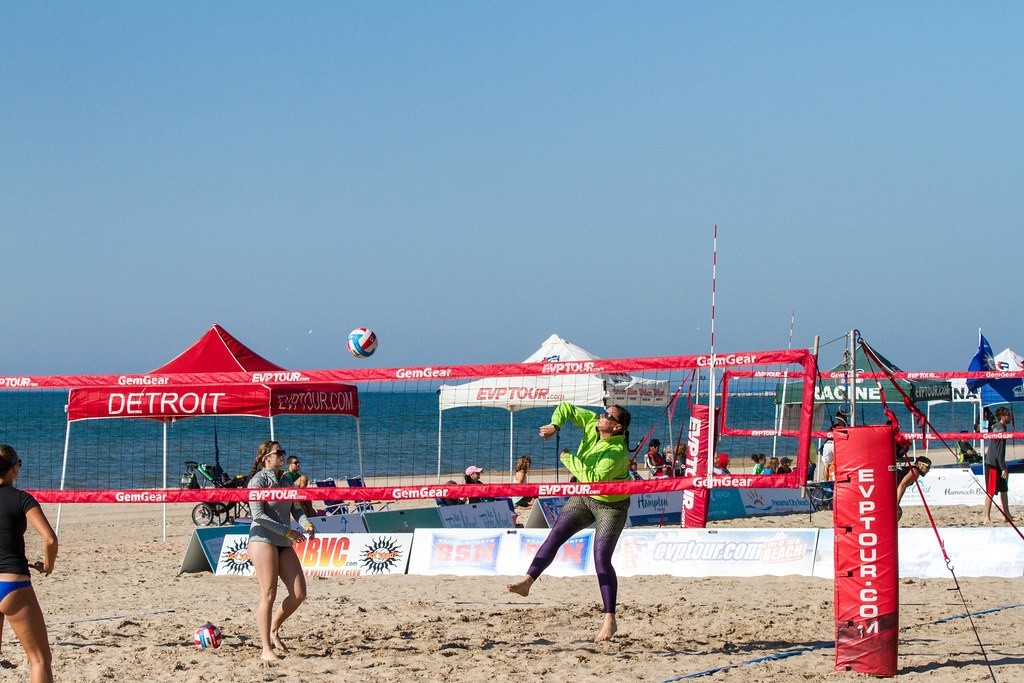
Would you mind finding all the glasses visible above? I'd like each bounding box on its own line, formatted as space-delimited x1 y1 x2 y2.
1004 412 1010 417
603 410 620 424
652 445 659 448
920 462 930 470
14 459 22 466
290 461 300 465
262 450 286 463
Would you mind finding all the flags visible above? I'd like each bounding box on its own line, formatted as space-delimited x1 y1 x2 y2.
966 334 995 391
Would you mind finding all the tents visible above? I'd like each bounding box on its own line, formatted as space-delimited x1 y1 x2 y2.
772 335 1024 472
53 324 363 544
432 336 677 505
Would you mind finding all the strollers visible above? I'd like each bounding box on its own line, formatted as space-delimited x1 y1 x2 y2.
190 462 252 528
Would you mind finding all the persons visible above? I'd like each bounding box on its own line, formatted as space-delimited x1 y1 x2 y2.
819 411 849 481
956 405 995 464
442 466 517 527
896 457 931 521
629 439 730 485
983 407 1021 524
281 455 312 517
509 455 538 508
750 452 816 481
245 441 306 660
504 402 632 643
0 444 58 683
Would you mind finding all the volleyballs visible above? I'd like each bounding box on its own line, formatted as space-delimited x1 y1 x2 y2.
193 624 222 649
346 328 378 359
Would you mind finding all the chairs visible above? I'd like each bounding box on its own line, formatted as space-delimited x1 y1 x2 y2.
341 475 394 512
957 442 981 471
644 453 674 479
308 477 349 515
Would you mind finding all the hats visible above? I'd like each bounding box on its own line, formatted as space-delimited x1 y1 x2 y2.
466 465 483 476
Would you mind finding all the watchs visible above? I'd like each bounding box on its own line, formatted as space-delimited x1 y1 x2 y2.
552 423 560 430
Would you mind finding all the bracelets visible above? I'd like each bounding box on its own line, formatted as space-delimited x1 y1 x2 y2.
305 524 313 527
285 529 293 537
